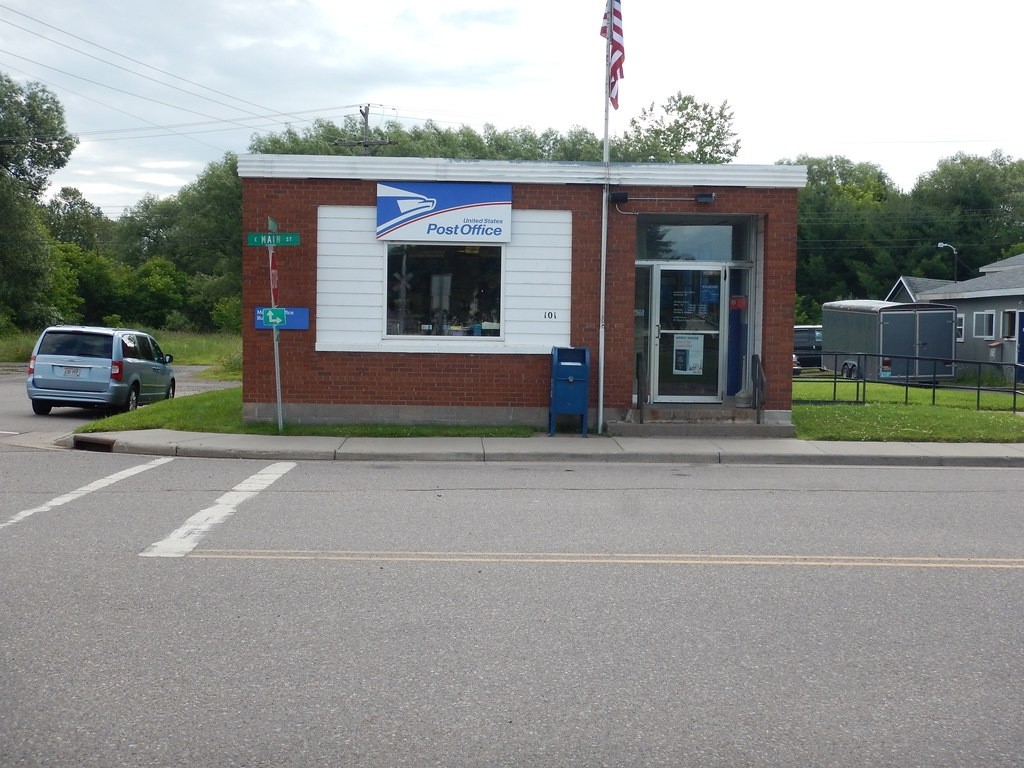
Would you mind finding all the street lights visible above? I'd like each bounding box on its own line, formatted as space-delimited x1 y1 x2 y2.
937 242 958 284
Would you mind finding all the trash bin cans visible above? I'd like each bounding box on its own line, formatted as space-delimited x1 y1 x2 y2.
548 346 590 438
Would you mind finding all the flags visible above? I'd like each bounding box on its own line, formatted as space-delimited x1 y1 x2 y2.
600 0 625 110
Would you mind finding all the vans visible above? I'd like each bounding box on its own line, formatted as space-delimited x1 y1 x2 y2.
793 325 824 369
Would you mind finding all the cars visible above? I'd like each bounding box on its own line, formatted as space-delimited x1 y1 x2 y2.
27 326 176 414
